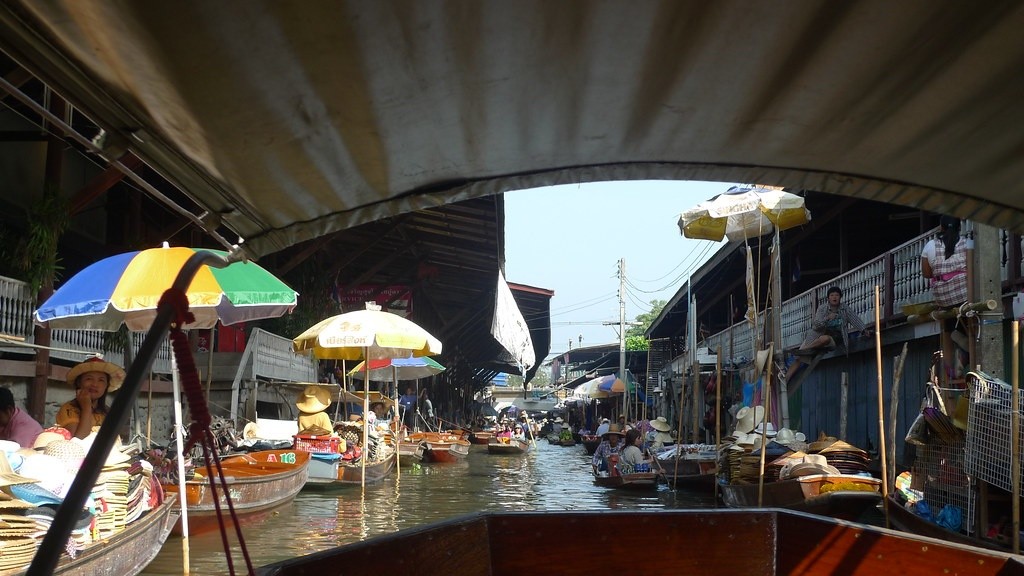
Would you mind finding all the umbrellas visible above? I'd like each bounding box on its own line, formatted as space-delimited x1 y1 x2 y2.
34 242 299 573
574 374 634 419
676 185 809 338
293 308 442 485
347 355 446 479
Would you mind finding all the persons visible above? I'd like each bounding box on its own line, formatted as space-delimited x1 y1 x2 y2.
398 387 434 433
922 215 968 351
578 415 673 475
0 387 44 449
482 416 572 446
801 288 871 361
295 386 334 435
369 402 400 431
56 357 125 440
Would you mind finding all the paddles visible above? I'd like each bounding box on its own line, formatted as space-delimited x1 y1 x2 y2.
523 409 538 450
650 450 674 491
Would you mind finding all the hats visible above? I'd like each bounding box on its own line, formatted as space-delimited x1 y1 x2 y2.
718 423 869 480
66 357 126 392
649 417 671 431
515 424 522 428
735 405 764 433
601 424 626 439
369 397 391 414
0 426 164 572
554 417 564 423
602 418 610 423
295 386 332 413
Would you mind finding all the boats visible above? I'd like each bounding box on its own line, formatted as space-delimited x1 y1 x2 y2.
398 444 427 466
157 449 312 538
0 488 183 576
307 421 399 487
716 472 884 529
886 492 1024 556
591 472 661 490
409 420 604 462
652 444 716 483
